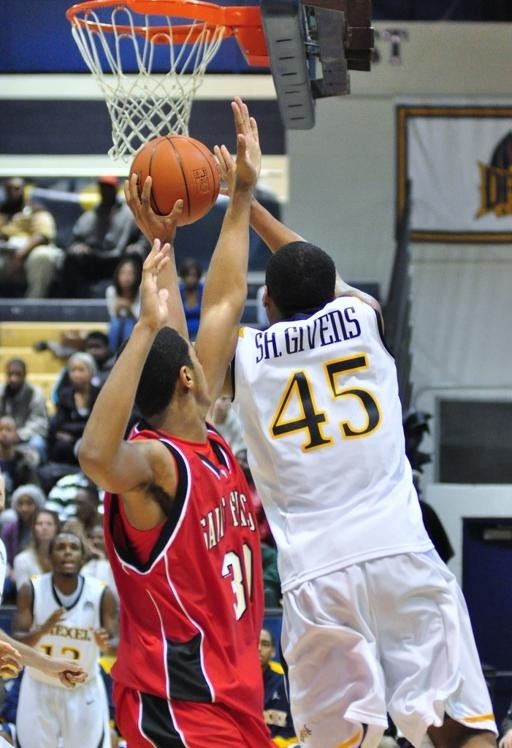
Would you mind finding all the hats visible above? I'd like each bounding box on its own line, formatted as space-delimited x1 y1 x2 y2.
10 483 46 512
66 351 101 388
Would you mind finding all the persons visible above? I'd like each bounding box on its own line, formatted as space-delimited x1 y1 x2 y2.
75 96 276 746
1 327 299 747
119 145 499 747
0 175 203 345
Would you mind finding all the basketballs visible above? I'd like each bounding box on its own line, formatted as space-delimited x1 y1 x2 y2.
128 134 222 226
